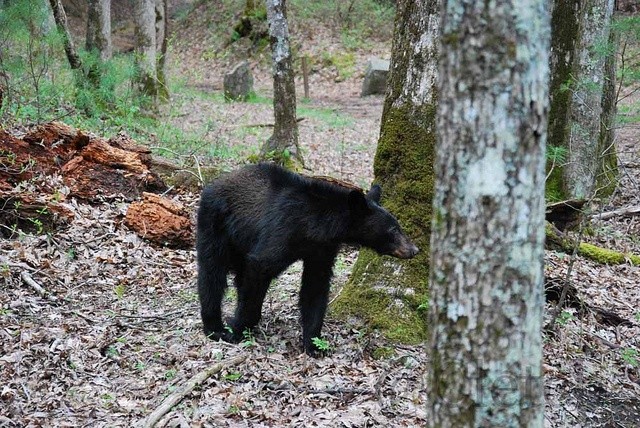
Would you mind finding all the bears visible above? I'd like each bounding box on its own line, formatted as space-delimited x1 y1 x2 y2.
197 163 421 358
